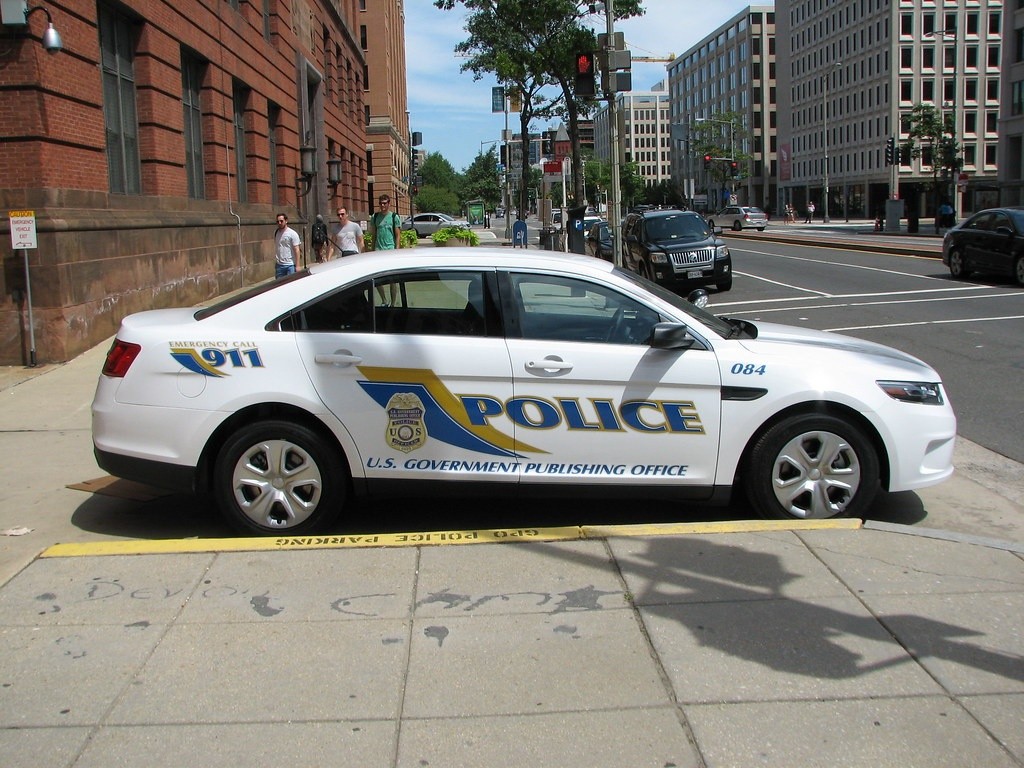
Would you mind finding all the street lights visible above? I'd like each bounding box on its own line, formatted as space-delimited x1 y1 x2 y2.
822 62 842 224
695 117 735 207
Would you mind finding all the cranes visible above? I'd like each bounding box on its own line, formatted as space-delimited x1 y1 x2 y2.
622 40 677 72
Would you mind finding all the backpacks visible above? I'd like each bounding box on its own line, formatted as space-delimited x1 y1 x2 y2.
314 223 324 244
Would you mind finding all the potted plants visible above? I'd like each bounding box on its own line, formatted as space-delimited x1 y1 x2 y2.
431 224 479 247
363 228 418 252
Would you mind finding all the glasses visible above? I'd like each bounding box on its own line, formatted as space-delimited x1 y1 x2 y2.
337 213 345 216
276 219 284 223
380 201 388 205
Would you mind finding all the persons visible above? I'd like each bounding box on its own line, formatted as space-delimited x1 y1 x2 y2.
370 194 402 307
272 213 301 278
783 204 789 224
804 201 815 223
311 206 364 261
789 203 797 223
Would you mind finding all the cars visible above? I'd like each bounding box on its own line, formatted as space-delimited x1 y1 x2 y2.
401 212 473 238
942 205 1024 289
704 205 768 232
87 248 959 537
550 206 613 263
934 204 954 228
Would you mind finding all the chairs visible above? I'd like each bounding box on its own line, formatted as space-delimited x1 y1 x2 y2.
466 276 543 338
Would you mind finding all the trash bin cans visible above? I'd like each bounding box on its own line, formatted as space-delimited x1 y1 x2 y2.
538 226 556 245
544 229 563 252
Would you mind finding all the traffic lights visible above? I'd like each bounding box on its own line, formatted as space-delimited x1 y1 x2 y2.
731 162 739 176
409 174 418 196
574 50 598 97
412 148 419 173
894 146 903 165
886 137 894 165
703 153 711 170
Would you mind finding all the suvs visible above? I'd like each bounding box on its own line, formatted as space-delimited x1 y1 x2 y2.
620 208 733 291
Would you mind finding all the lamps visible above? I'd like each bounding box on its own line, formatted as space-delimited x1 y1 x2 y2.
296 131 318 197
326 138 342 201
24 5 64 56
